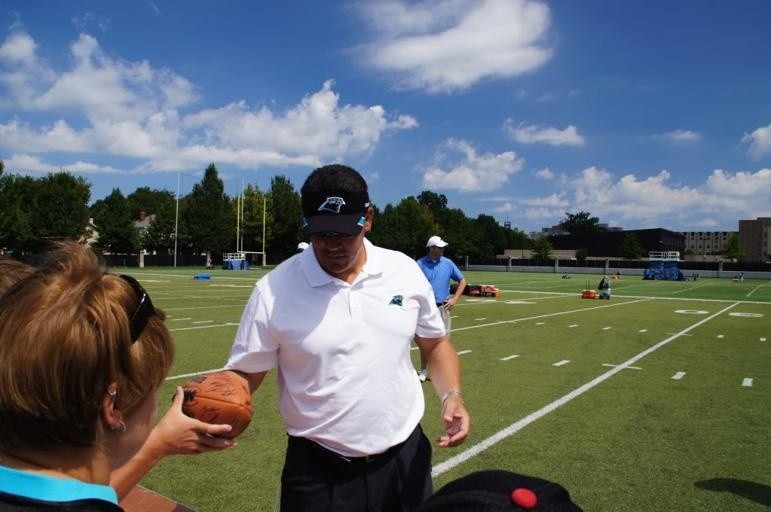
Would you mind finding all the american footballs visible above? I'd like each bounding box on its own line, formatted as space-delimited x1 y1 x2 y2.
172 372 253 440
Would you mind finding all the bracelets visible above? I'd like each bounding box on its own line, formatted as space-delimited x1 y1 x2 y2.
442 390 464 401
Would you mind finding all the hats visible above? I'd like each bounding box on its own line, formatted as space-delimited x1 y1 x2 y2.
426 236 448 248
300 195 370 236
416 471 581 511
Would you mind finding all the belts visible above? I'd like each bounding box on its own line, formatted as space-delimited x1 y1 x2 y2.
436 302 447 307
344 455 379 465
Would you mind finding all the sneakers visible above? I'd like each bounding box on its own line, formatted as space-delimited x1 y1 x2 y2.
419 370 428 382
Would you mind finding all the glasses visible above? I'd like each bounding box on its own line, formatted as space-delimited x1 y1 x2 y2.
119 274 156 343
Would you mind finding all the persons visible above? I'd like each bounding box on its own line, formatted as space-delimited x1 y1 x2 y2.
186 158 470 512
0 258 235 501
0 232 173 512
417 235 469 384
